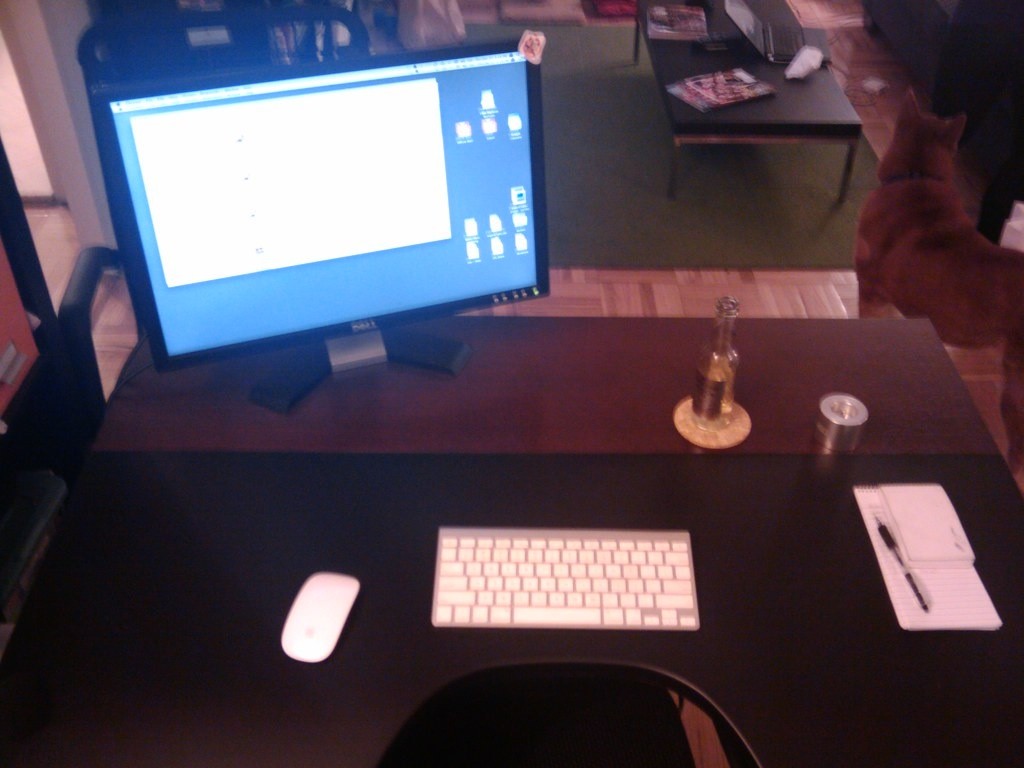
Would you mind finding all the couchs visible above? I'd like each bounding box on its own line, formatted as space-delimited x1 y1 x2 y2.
863 0 1024 245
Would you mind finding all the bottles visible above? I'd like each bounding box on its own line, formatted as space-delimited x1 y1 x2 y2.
693 296 740 430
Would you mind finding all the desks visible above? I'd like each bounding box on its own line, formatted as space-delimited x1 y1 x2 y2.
634 1 863 202
0 314 1024 768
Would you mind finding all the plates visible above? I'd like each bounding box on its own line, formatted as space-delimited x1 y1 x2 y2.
674 398 752 450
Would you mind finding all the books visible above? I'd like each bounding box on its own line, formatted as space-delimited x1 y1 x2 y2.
646 3 707 42
666 67 777 113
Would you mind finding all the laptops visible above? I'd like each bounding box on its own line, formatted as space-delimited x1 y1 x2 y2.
723 0 831 65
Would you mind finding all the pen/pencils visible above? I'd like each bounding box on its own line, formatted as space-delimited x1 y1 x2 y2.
873 511 932 616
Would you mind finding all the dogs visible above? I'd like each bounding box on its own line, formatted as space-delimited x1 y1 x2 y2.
853 84 1024 483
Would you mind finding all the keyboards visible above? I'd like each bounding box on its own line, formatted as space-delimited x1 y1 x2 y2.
431 527 700 629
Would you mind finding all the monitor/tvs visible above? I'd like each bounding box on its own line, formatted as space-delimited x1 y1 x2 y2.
88 36 550 414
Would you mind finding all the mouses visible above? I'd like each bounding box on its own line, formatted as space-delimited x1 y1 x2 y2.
280 571 361 663
